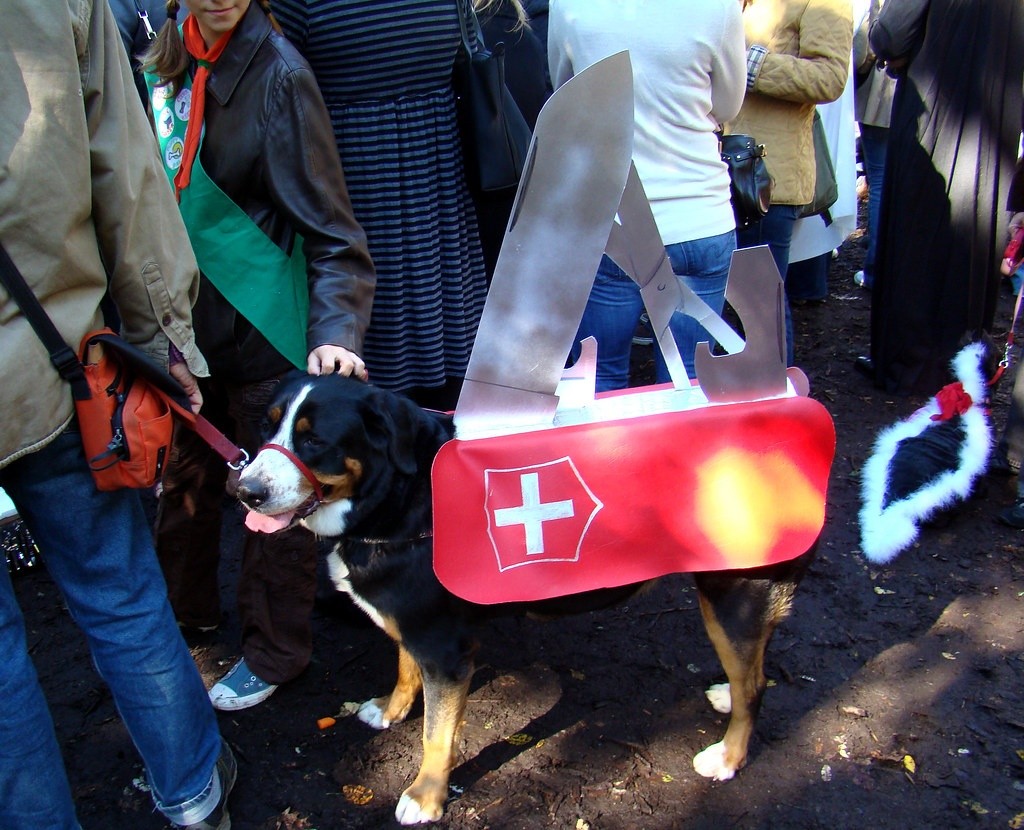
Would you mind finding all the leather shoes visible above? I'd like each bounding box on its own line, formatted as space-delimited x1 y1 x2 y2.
169 738 237 830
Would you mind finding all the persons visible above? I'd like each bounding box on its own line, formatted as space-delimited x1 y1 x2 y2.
853 0 1024 530
0 0 854 830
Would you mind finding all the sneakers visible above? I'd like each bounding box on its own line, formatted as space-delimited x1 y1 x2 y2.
208 657 278 710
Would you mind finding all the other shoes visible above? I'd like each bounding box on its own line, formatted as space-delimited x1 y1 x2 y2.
854 270 866 288
855 355 877 379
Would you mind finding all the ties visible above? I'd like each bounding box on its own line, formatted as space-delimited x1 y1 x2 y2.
171 13 230 201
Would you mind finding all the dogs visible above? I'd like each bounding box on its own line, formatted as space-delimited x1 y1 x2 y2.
235 372 822 825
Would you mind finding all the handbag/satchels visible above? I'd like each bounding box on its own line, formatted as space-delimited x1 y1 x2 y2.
714 132 774 231
455 0 534 189
73 328 191 490
129 52 147 87
